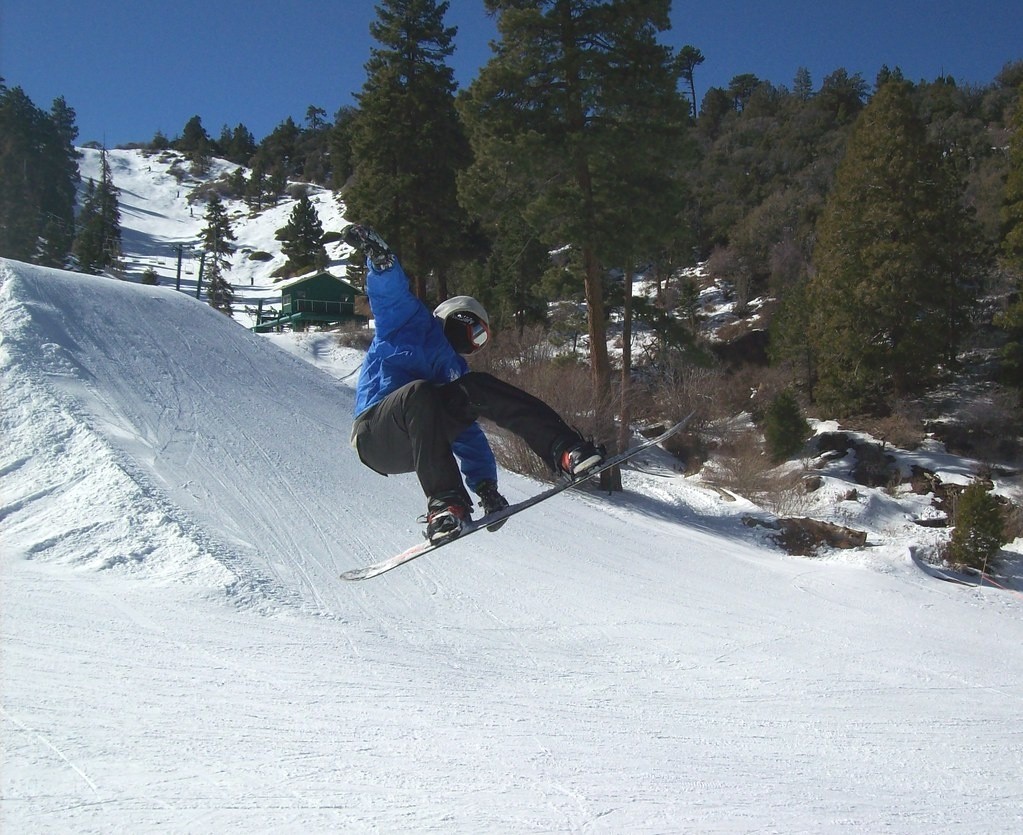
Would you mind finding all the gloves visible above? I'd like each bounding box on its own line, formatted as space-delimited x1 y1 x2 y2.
476 481 511 532
341 222 395 274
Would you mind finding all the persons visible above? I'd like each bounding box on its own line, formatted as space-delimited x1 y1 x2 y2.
346 238 605 549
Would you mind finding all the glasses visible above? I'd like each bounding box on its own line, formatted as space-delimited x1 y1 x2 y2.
451 304 487 351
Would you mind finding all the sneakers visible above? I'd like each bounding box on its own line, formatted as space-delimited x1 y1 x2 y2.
551 435 608 479
418 497 471 547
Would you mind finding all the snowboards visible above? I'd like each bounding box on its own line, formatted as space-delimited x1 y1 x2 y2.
341 407 698 581
